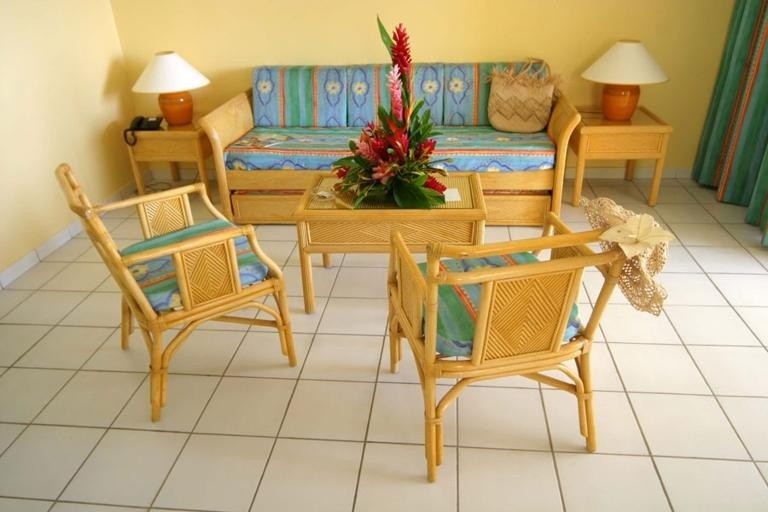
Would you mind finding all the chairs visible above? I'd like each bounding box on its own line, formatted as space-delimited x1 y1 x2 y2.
384 213 632 484
56 164 298 423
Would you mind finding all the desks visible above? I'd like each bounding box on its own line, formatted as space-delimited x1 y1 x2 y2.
292 172 490 315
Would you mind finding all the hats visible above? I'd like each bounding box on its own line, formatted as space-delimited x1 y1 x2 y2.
584 197 669 316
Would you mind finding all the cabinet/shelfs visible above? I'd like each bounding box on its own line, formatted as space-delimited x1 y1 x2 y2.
122 114 214 199
567 99 674 214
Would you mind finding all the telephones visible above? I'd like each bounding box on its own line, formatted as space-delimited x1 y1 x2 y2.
123 115 163 147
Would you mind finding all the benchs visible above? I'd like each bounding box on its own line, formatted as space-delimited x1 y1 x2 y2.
196 58 581 232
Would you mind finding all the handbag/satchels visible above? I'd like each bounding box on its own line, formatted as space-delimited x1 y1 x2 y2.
487 67 559 133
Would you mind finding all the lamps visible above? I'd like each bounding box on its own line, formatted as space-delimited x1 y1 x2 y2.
582 34 671 120
129 48 211 123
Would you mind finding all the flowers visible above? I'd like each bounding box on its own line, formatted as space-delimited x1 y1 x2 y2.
324 10 451 213
